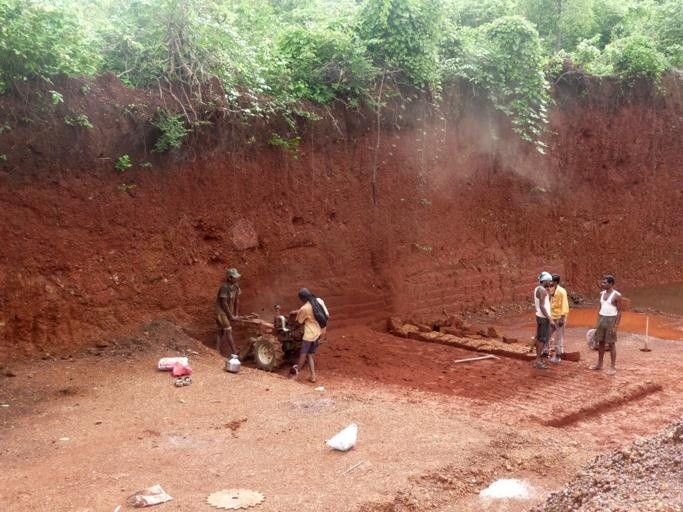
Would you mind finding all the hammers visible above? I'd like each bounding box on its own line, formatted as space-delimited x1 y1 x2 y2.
639 316 651 352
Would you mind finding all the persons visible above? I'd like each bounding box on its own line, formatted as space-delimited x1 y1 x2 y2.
214 268 243 355
528 274 569 363
533 272 560 368
296 288 329 383
589 275 622 375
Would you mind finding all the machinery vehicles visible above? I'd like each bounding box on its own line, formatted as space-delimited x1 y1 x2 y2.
229 303 324 370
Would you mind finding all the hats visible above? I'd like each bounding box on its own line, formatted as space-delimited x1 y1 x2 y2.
227 269 240 278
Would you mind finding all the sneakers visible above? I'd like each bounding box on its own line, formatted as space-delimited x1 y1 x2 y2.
550 357 561 363
182 376 191 386
175 377 183 387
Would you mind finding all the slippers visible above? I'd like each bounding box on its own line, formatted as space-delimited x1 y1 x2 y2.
536 363 546 369
606 368 615 374
